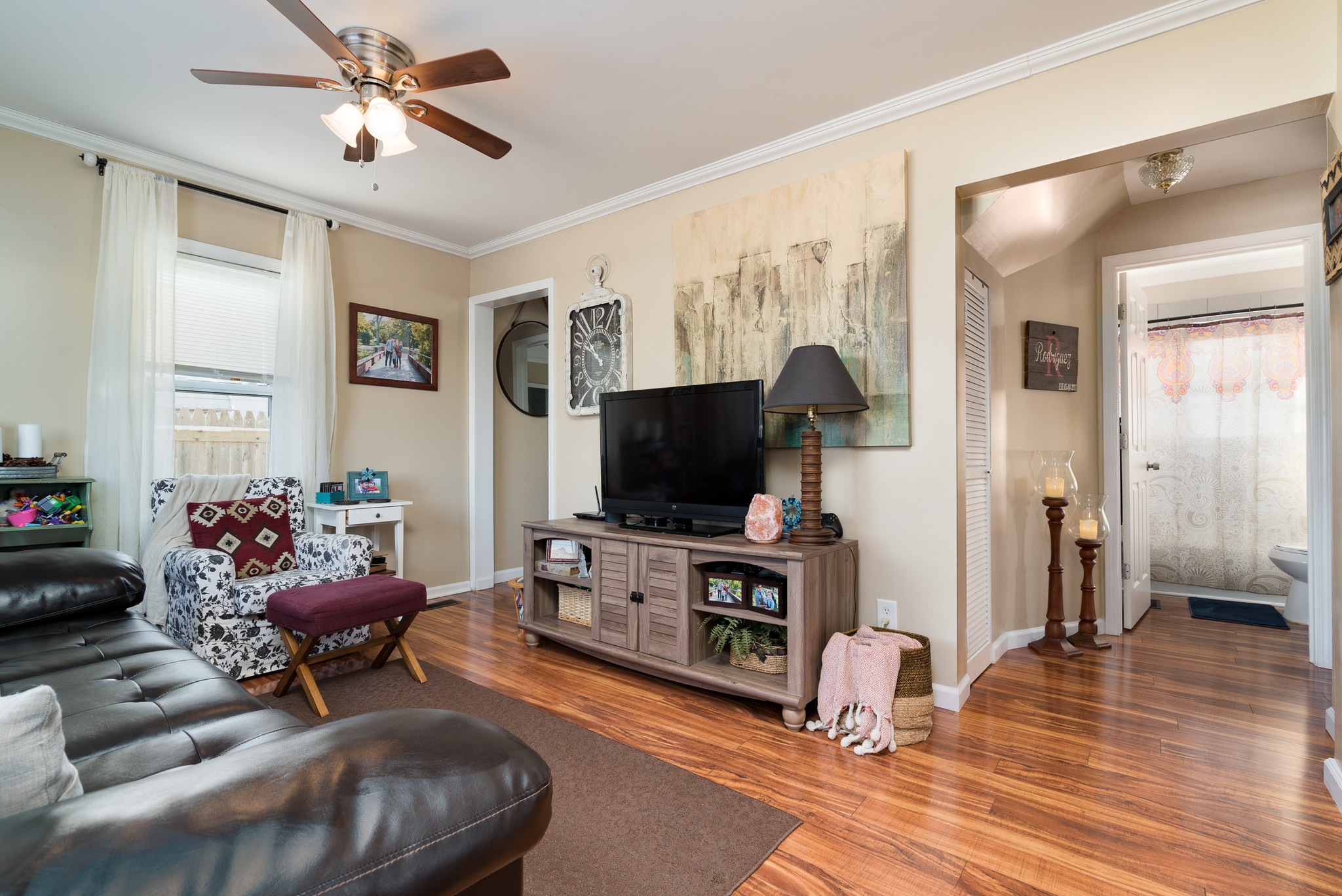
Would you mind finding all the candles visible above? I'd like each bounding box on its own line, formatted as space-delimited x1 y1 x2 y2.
17 421 42 458
1046 477 1097 540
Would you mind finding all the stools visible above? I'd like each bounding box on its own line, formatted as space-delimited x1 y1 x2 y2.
266 574 427 717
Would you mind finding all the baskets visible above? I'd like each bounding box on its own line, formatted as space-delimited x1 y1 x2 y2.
557 584 592 628
730 641 788 674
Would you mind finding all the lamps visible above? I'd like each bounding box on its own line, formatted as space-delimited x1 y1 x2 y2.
1138 148 1194 196
762 342 869 546
320 83 417 191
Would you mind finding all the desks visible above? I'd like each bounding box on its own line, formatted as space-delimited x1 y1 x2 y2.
306 498 413 579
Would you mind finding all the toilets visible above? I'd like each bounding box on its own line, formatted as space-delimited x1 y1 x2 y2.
1267 543 1309 625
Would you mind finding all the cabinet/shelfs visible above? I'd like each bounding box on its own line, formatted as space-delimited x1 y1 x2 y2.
517 517 858 732
0 476 97 547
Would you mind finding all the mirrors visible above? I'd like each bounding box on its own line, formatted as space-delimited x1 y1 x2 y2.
496 321 550 417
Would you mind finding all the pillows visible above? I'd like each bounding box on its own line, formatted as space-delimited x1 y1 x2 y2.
186 494 298 580
0 684 84 817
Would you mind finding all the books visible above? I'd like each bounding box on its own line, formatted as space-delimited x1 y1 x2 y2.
516 577 524 621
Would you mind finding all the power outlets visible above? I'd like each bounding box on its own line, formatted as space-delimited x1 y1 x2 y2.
877 599 898 630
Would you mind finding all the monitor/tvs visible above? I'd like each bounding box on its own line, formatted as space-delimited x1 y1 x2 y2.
599 380 766 537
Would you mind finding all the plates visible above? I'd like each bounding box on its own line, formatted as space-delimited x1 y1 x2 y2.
334 500 360 505
367 499 392 502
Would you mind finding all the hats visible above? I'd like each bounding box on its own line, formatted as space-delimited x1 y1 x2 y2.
393 337 396 340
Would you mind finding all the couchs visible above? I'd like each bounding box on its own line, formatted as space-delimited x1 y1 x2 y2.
0 477 552 896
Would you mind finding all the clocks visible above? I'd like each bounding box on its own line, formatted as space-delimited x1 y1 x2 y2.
565 253 633 416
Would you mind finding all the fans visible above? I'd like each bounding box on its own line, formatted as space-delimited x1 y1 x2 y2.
190 0 512 162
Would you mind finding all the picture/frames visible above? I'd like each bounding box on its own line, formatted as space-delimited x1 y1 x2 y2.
320 467 389 501
349 302 439 391
704 572 786 620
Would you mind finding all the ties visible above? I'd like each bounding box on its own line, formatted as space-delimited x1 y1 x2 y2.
721 584 722 589
391 341 393 351
760 593 763 605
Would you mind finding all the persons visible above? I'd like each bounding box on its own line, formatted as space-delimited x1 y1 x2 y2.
755 583 775 611
717 580 729 600
332 486 341 492
355 479 380 493
384 337 403 369
578 549 589 576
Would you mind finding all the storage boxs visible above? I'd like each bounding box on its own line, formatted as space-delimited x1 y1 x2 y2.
315 491 345 504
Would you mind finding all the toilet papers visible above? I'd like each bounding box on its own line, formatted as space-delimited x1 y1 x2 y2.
18 424 43 458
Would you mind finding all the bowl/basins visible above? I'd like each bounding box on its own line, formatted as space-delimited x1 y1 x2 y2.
7 508 37 526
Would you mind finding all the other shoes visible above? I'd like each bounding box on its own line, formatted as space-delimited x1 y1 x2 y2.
396 366 397 368
394 366 395 369
389 366 392 368
384 366 386 368
399 366 401 368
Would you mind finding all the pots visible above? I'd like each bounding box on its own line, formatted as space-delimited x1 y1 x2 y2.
0 452 67 479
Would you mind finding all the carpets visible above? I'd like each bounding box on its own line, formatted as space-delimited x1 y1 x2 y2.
255 657 804 896
1187 597 1291 630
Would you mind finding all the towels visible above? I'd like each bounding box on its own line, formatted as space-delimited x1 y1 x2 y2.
131 473 252 632
